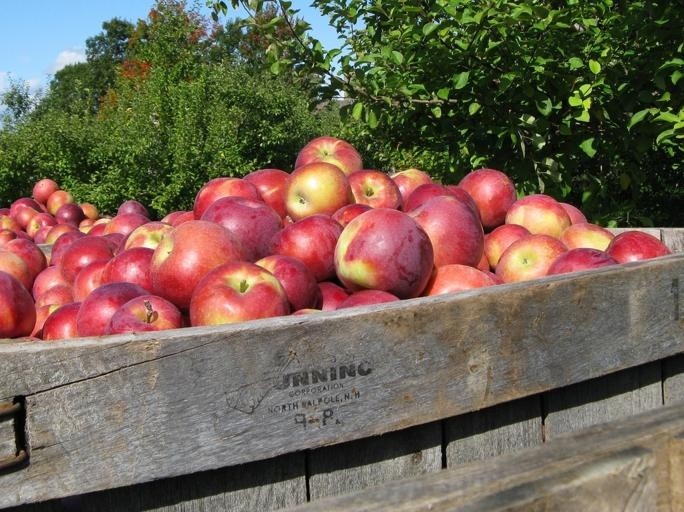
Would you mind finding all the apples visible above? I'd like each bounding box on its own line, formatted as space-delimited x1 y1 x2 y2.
0 137 672 340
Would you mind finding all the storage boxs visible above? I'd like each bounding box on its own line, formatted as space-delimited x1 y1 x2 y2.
0 221 683 510
